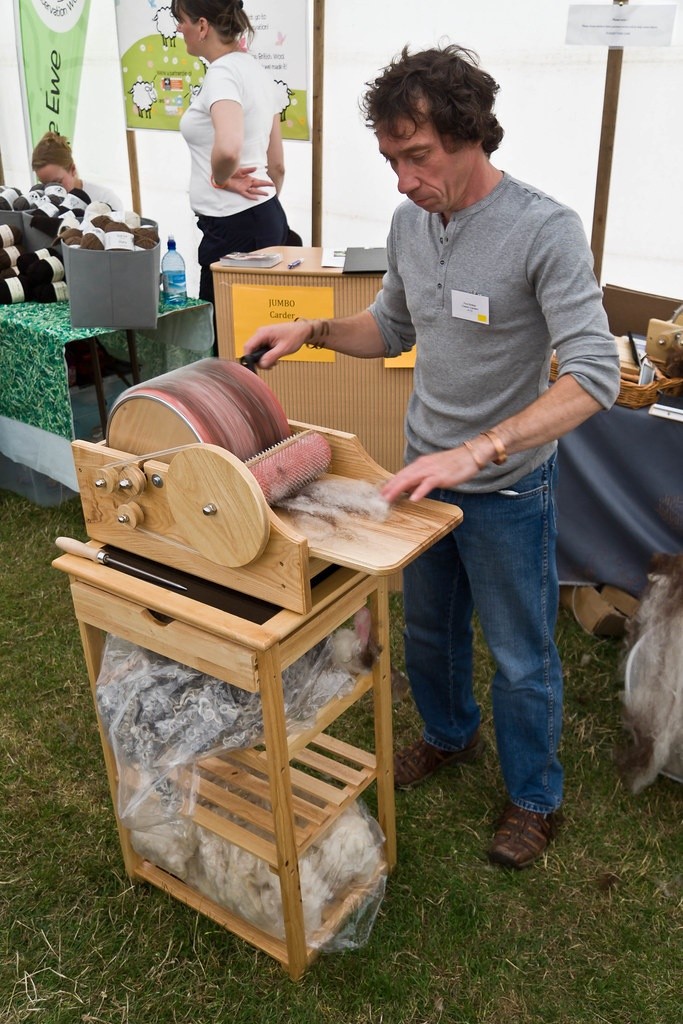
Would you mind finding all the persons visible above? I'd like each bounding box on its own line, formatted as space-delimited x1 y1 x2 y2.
31 132 121 212
240 42 621 873
169 0 302 354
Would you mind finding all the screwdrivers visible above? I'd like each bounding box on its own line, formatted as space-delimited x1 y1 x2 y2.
54 536 188 591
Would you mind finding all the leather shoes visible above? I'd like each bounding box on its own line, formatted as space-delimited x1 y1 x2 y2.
389 731 554 865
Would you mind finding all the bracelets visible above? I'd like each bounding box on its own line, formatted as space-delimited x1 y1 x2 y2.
295 315 331 354
209 170 227 190
462 437 485 472
479 428 509 465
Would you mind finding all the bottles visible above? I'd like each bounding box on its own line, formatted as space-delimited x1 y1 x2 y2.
162 233 187 308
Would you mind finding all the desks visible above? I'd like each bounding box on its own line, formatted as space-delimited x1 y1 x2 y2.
1 244 212 505
550 333 683 591
211 245 416 476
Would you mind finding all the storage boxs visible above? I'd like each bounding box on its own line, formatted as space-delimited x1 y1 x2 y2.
0 180 162 331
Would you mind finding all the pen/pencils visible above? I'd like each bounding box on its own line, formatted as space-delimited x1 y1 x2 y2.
287 258 303 269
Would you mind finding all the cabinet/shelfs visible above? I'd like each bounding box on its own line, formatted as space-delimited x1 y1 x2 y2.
48 538 400 981
